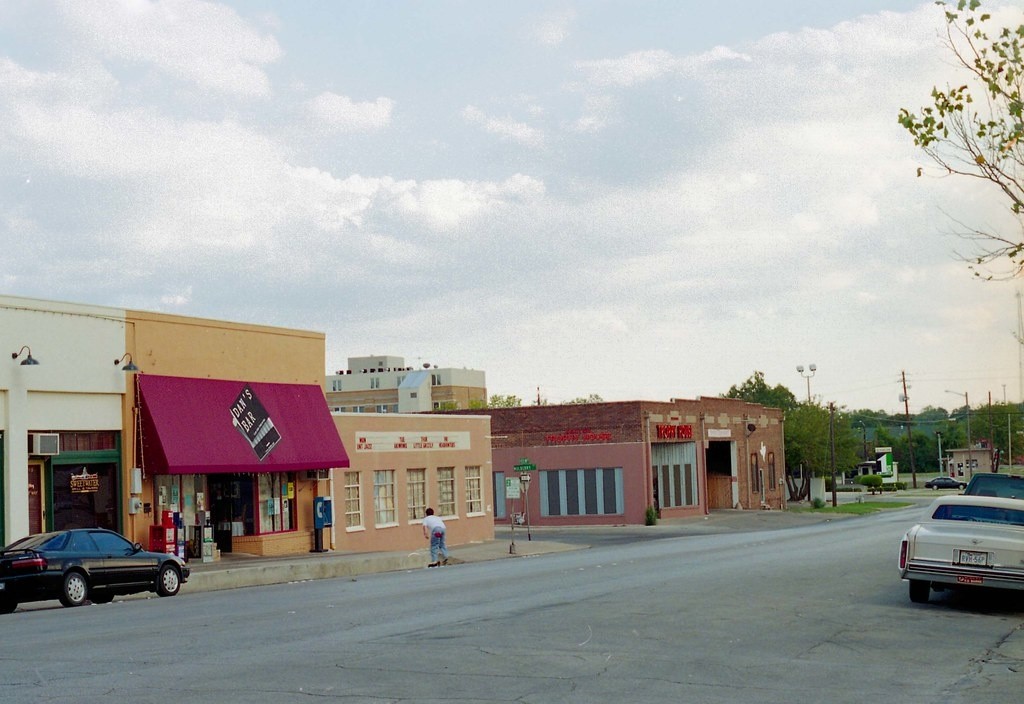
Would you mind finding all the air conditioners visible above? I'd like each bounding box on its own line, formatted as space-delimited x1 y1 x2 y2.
301 470 330 481
28 433 60 456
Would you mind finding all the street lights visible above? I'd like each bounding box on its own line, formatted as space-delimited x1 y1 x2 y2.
797 364 816 404
944 390 973 479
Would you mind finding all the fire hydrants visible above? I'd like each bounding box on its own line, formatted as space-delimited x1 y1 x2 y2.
859 496 864 503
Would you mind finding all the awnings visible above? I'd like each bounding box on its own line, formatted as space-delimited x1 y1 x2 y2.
134 373 350 473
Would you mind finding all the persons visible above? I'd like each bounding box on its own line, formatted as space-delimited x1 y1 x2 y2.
423 508 448 567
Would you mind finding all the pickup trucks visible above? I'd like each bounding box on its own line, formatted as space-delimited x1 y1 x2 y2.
952 473 1024 526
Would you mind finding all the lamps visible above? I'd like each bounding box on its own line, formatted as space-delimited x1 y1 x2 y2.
12 346 39 366
114 354 139 371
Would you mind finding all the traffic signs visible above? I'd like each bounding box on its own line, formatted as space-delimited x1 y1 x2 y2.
520 474 530 483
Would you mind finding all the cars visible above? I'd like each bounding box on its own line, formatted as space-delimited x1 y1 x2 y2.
898 496 1024 604
0 528 191 615
924 477 969 490
